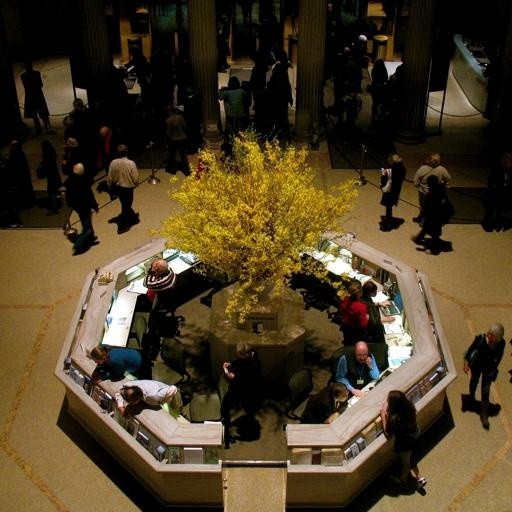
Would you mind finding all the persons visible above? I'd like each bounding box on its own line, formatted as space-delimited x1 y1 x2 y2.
40 140 62 215
59 100 139 233
221 340 262 427
338 280 370 346
381 155 406 230
90 344 150 383
21 63 50 133
68 163 100 246
217 2 296 150
360 281 395 342
464 323 505 429
485 56 512 158
109 42 203 175
413 153 450 245
143 259 178 310
482 153 512 232
2 139 32 227
323 1 406 158
113 379 183 416
302 382 351 424
332 341 380 397
380 390 426 490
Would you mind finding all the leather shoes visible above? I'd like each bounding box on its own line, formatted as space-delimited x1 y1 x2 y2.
482 423 490 431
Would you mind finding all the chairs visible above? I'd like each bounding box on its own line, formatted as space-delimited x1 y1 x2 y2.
151 337 193 406
128 294 160 352
289 369 313 420
190 367 232 449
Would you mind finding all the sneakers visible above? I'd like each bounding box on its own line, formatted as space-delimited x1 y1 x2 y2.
393 478 407 484
416 478 427 491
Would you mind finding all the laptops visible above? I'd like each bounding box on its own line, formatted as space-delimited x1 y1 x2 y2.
380 291 403 316
